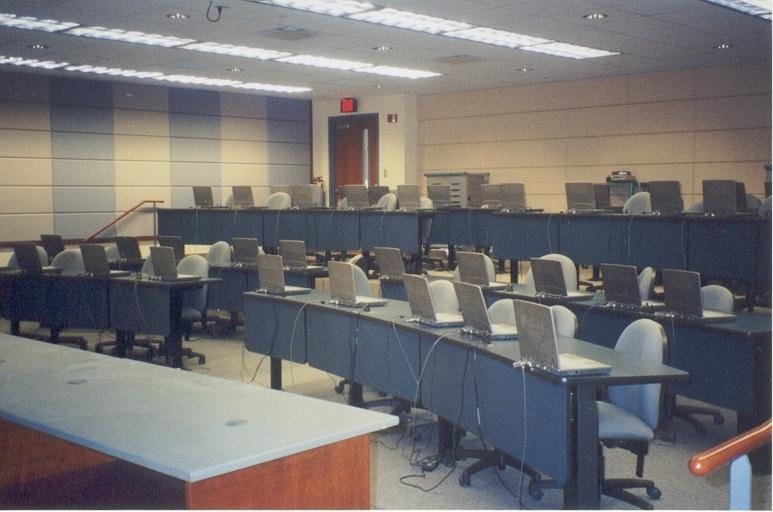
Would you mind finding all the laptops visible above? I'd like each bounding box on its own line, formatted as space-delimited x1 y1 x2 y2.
326 261 388 307
452 281 519 340
374 246 409 282
278 240 324 272
529 257 594 301
511 298 613 378
401 273 464 328
39 234 66 266
192 186 228 208
231 237 259 267
480 183 544 211
14 242 64 274
455 251 514 290
231 186 268 208
271 184 392 211
150 246 202 281
426 185 461 207
158 236 186 266
599 262 666 311
80 244 133 277
397 184 435 213
115 236 147 264
565 181 761 215
663 268 737 323
256 254 313 295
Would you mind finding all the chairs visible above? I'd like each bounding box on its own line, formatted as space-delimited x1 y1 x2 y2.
0 178 770 509
